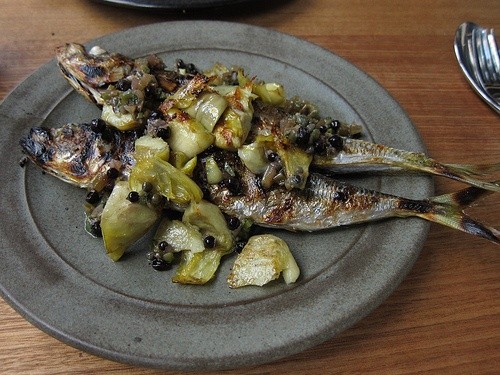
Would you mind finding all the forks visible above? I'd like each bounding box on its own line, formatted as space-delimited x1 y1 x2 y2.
472 26 500 106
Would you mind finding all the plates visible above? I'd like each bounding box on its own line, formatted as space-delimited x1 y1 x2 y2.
96 0 292 15
0 18 436 372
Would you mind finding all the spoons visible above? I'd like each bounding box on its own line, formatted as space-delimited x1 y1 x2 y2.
453 20 500 114
